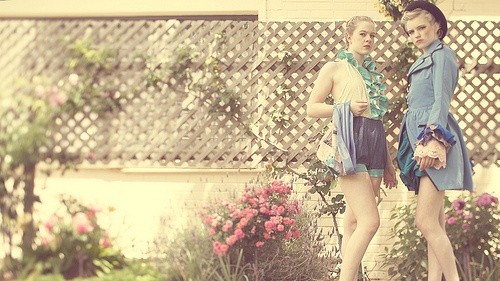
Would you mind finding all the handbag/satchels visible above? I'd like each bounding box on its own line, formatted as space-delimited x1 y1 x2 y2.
316 115 348 176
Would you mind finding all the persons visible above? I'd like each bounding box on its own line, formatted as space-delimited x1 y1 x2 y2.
399 1 473 281
307 16 398 281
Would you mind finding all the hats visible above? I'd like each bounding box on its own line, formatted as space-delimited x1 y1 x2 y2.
402 0 447 39
